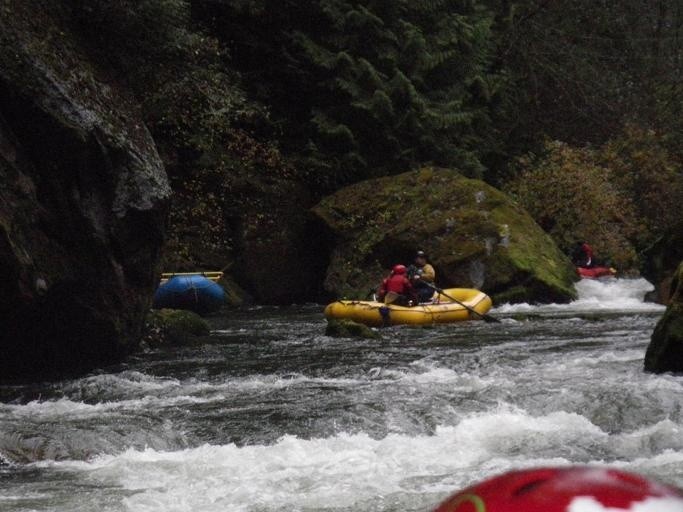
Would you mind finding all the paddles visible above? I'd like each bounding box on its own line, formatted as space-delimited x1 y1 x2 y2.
417 279 500 323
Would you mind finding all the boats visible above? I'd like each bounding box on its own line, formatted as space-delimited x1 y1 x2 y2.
155 273 223 312
576 267 619 281
325 287 492 326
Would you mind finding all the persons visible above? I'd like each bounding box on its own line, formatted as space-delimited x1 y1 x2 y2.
378 264 418 308
571 239 594 269
406 249 436 302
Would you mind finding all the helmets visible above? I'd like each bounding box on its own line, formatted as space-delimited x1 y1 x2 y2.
412 251 428 258
430 467 683 512
392 264 407 274
